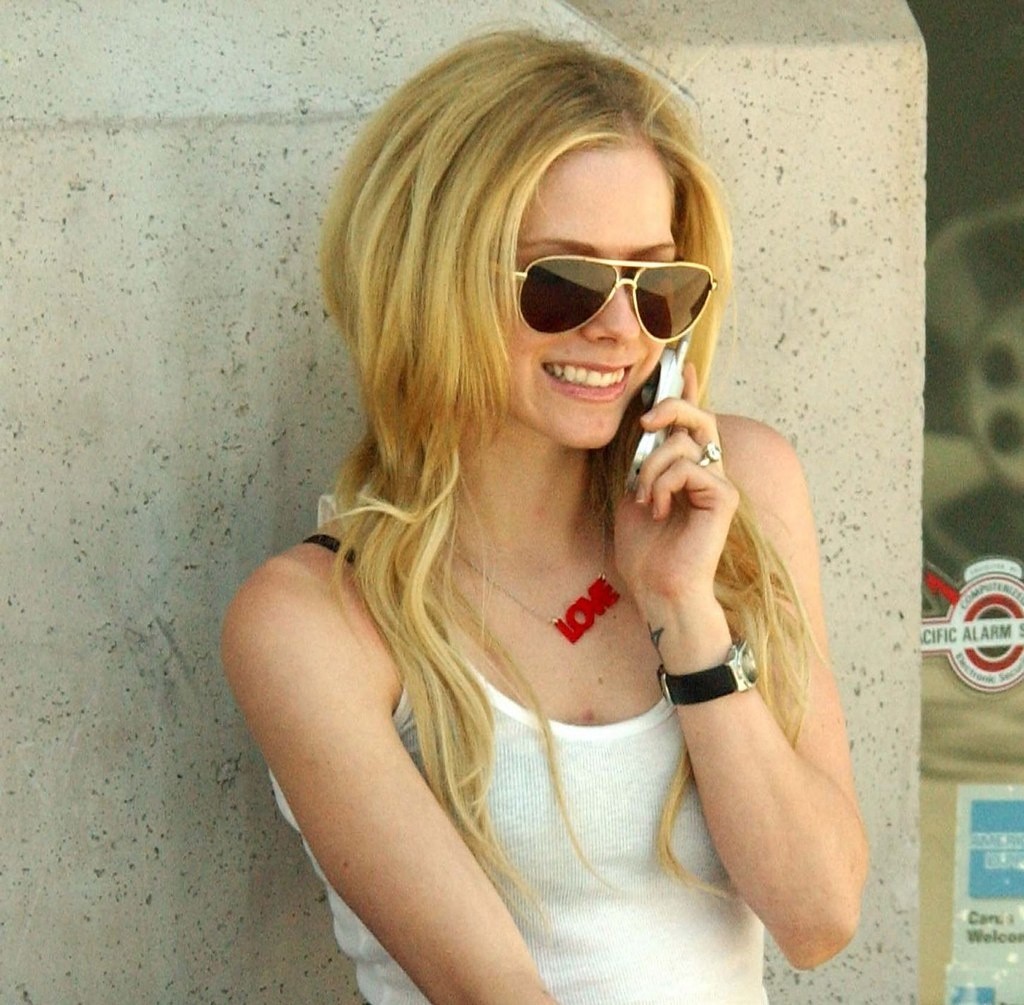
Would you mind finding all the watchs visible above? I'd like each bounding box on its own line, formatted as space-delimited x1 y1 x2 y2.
657 640 759 706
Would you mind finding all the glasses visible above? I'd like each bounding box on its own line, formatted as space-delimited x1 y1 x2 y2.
514 255 719 344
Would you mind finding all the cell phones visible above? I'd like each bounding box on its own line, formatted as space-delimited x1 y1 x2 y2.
624 330 693 493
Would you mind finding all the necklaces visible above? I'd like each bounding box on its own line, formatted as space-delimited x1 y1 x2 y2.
446 516 620 644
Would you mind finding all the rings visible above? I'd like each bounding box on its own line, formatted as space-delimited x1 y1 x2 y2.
698 441 720 465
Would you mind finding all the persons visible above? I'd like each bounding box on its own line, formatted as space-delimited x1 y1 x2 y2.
224 25 870 1005
